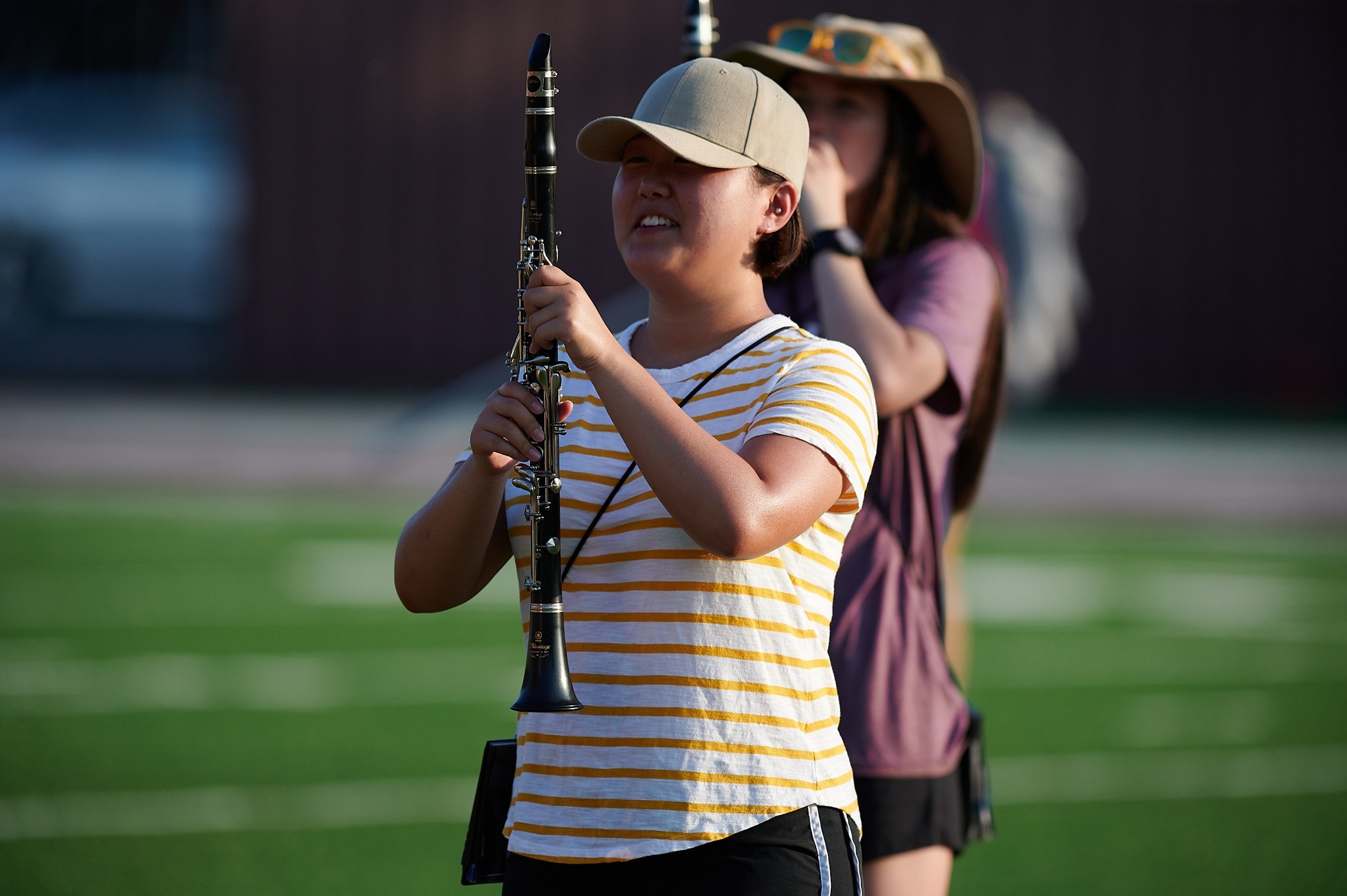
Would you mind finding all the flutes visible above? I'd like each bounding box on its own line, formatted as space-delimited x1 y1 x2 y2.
508 33 587 713
678 0 720 65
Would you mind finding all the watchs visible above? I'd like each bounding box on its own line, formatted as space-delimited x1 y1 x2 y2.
802 225 861 263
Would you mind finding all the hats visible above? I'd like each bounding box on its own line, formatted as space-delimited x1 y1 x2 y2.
575 57 810 199
731 13 982 221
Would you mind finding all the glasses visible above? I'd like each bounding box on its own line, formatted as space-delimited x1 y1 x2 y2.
767 20 915 81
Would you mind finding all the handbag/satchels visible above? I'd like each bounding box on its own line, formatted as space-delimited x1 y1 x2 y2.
458 736 522 887
955 706 998 845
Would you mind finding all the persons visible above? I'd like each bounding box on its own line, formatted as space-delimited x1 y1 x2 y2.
729 16 1007 895
393 57 878 894
